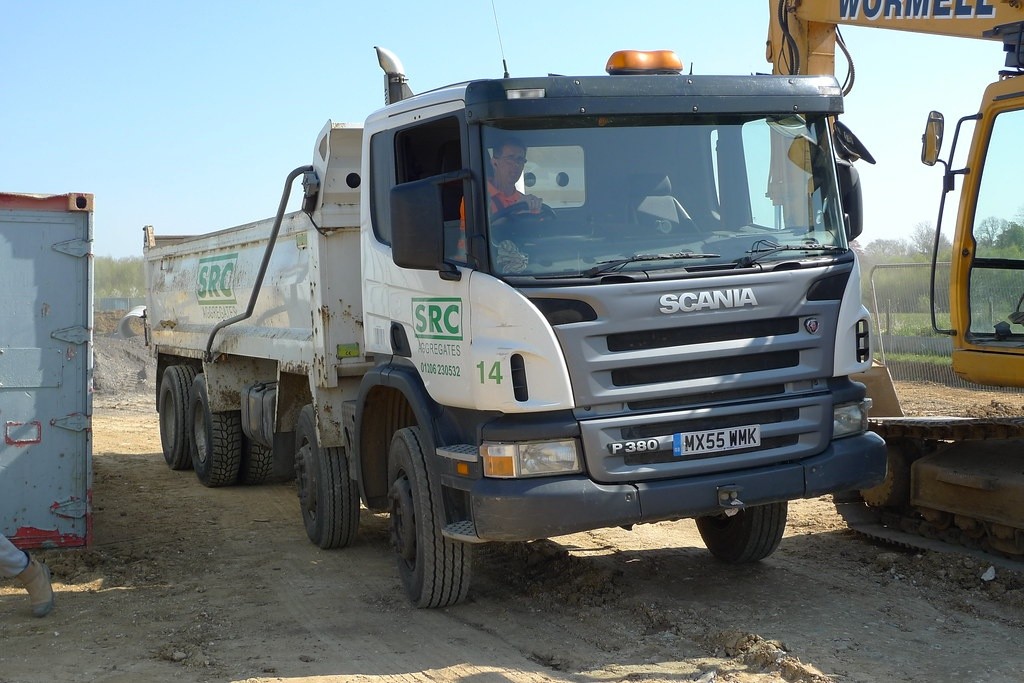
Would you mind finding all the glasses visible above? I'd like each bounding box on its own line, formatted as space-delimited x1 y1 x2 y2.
493 155 527 164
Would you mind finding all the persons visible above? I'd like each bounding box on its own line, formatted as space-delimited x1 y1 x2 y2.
0 532 54 618
456 136 545 263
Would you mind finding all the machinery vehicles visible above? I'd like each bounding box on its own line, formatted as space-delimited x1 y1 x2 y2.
763 0 1023 582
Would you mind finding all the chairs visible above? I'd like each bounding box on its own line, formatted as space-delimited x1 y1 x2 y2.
628 172 701 234
427 139 494 220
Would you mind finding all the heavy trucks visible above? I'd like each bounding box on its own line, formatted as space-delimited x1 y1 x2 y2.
141 43 888 610
